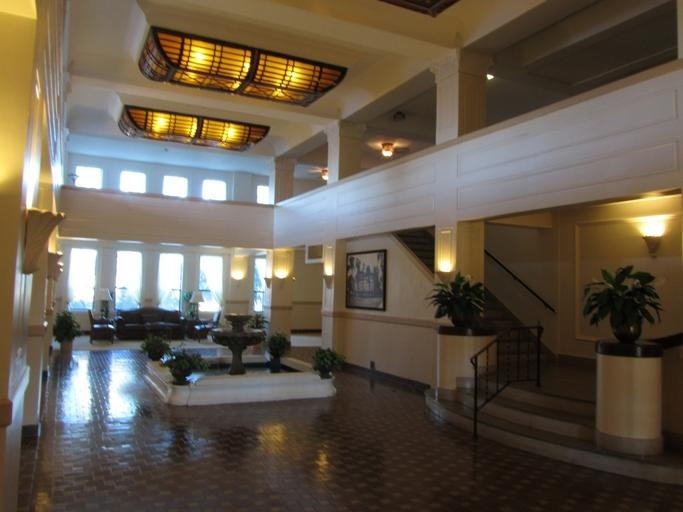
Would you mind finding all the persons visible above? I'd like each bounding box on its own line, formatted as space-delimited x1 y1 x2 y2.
347 253 383 299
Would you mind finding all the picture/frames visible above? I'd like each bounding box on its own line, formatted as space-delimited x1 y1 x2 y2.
345 249 386 311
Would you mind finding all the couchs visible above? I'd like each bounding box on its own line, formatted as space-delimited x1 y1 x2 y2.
114 307 185 340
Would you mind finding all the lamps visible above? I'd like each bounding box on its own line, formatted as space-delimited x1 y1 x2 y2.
21 208 66 280
434 270 452 280
116 103 268 152
189 292 204 320
320 170 327 181
322 275 331 288
381 143 394 158
93 287 112 319
642 235 663 257
138 26 348 110
231 275 288 289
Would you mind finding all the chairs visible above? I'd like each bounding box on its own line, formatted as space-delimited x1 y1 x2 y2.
87 309 115 343
193 311 221 342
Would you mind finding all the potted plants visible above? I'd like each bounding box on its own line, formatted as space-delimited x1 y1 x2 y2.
246 312 270 354
423 274 496 390
140 334 170 361
52 312 79 354
583 262 665 458
267 332 291 372
310 345 345 380
160 347 199 385
